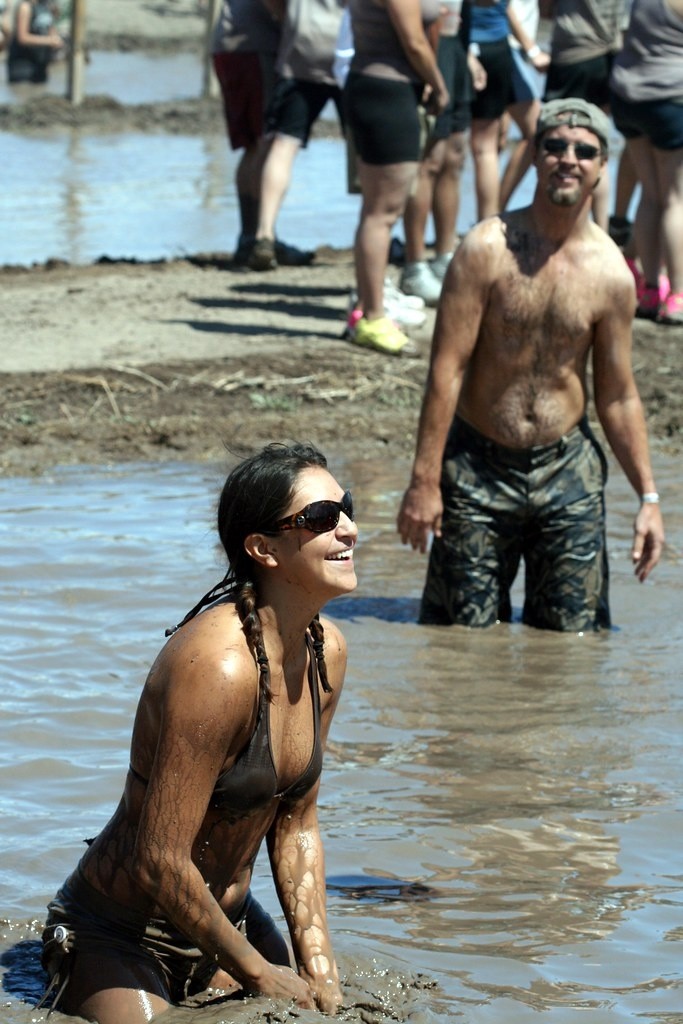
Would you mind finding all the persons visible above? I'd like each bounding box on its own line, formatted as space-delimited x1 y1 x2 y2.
0 0 683 634
43 441 358 1024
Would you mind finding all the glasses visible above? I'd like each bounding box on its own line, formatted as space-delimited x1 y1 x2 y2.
542 138 604 159
260 488 353 535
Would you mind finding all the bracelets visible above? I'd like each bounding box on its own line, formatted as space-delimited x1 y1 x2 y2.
638 492 660 505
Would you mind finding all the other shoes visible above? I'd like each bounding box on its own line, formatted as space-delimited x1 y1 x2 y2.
636 288 682 324
349 316 422 359
399 261 444 305
348 276 428 330
248 233 277 271
233 231 316 268
609 216 633 246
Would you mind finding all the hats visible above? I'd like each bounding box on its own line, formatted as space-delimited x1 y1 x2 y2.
535 97 611 160
